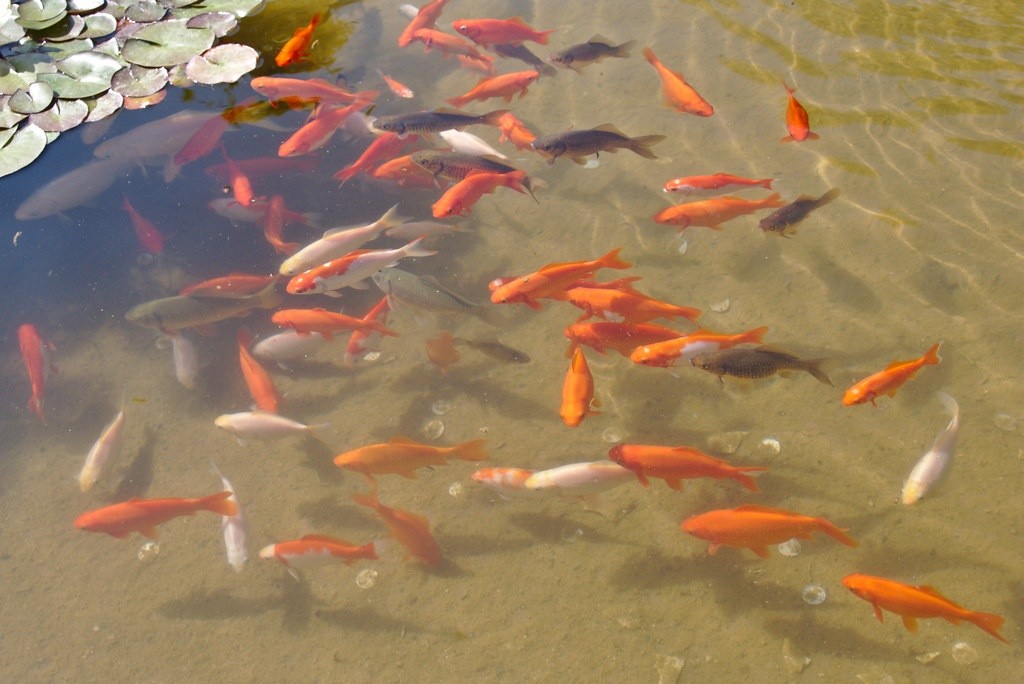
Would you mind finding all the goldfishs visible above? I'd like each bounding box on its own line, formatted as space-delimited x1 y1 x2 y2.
208 460 250 575
607 443 767 494
843 574 1011 646
465 465 538 498
236 329 284 414
70 489 241 544
17 322 61 429
12 0 840 428
77 399 133 491
522 460 635 498
334 433 490 484
840 342 943 407
356 492 440 571
682 505 857 560
258 535 390 581
214 410 336 445
900 389 960 506
